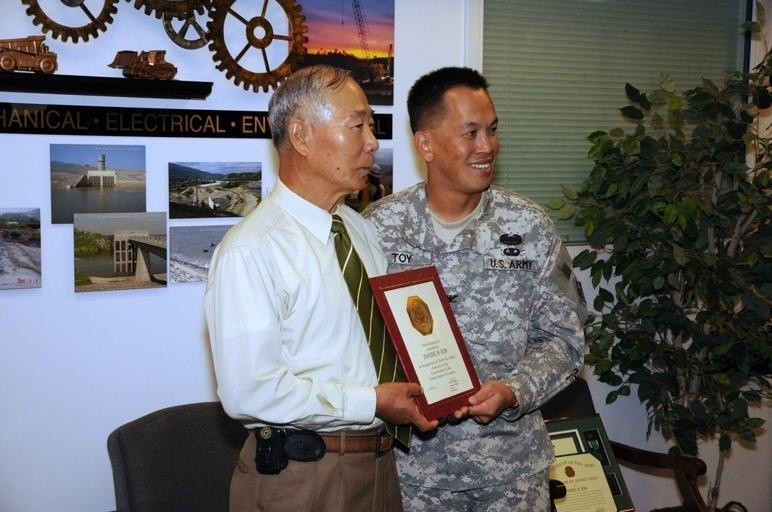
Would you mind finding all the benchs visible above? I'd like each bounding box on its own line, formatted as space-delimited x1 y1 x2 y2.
106 376 707 511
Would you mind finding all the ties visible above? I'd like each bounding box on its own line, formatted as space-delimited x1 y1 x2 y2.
331 214 414 450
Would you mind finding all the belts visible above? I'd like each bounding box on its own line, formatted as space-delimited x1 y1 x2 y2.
318 425 396 459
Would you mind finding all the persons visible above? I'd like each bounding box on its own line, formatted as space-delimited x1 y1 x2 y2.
356 67 588 511
203 65 445 511
